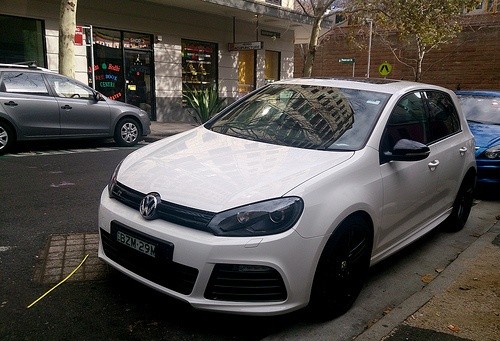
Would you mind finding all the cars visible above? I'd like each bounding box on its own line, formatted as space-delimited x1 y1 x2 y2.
0 59 152 155
93 75 477 326
455 88 500 196
